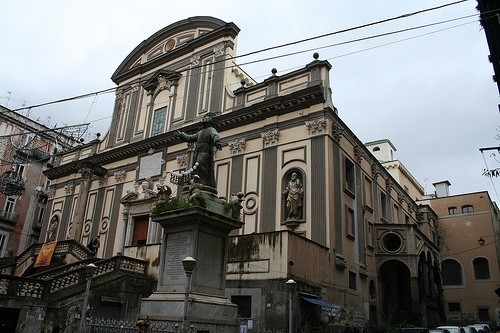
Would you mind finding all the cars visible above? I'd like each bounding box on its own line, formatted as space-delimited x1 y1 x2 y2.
425 322 496 333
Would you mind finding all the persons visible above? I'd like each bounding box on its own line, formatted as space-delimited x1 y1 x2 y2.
88 235 101 255
230 192 245 214
175 112 222 194
187 174 202 206
281 173 304 221
157 184 170 205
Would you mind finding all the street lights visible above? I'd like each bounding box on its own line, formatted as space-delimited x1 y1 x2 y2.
181 256 195 333
78 263 96 333
286 278 296 333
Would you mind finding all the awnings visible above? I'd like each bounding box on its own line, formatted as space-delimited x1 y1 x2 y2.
301 295 342 311
